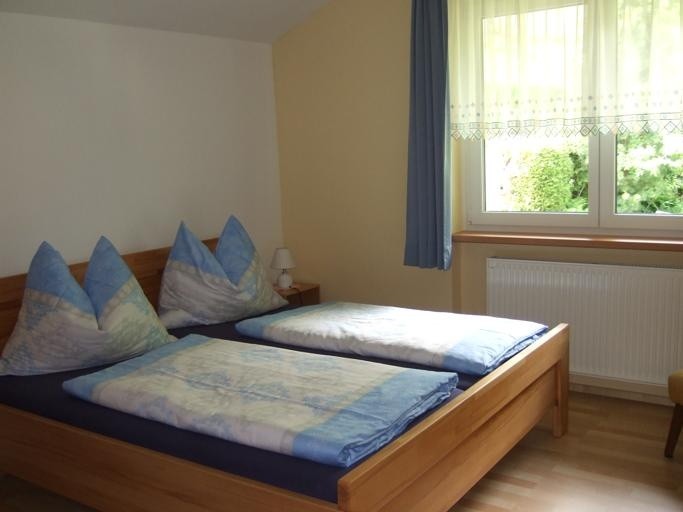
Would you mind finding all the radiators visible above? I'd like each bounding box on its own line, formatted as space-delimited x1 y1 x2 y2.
483 254 683 391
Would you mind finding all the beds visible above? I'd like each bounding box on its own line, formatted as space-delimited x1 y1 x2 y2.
0 237 572 511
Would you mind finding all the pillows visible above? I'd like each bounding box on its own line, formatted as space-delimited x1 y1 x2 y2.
155 217 290 334
0 235 188 378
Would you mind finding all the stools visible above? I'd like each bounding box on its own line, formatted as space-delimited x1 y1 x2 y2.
663 369 683 459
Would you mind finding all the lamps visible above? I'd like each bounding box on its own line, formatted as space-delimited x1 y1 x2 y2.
270 245 296 291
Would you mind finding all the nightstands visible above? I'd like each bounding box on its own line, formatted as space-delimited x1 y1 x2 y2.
273 281 322 305
